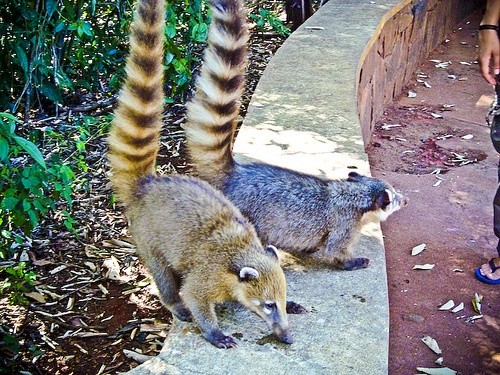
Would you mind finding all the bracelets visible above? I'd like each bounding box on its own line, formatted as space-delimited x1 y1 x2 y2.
478 24 498 31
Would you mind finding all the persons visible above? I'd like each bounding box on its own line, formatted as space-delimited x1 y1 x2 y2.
473 0 500 285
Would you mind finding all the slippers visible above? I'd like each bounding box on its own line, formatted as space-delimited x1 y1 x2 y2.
474 257 500 285
